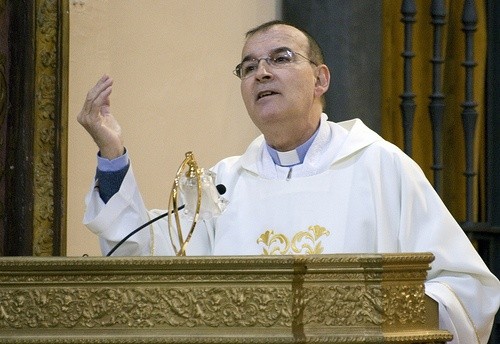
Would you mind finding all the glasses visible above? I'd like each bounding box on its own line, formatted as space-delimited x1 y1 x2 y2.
233 48 319 79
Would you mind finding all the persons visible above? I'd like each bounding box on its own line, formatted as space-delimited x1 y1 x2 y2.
76 21 500 344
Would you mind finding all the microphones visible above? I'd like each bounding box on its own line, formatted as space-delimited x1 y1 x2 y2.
106 184 227 257
